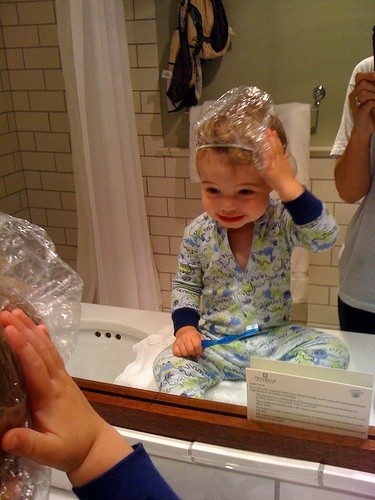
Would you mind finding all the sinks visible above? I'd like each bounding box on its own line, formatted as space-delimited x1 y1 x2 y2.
42 319 149 386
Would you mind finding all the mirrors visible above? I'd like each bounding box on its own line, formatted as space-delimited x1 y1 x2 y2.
1 0 375 476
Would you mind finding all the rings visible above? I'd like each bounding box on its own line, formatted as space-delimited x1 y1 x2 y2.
354 94 363 106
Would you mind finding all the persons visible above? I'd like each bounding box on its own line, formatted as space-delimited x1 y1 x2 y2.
0 279 181 500
152 87 350 399
328 56 374 334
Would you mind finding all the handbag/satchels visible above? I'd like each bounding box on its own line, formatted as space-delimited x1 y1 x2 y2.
166 27 203 107
188 0 232 61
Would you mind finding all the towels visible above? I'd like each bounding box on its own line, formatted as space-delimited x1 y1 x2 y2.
188 100 311 301
115 325 176 393
164 0 232 113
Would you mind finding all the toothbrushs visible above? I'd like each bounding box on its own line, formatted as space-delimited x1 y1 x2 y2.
192 324 260 349
